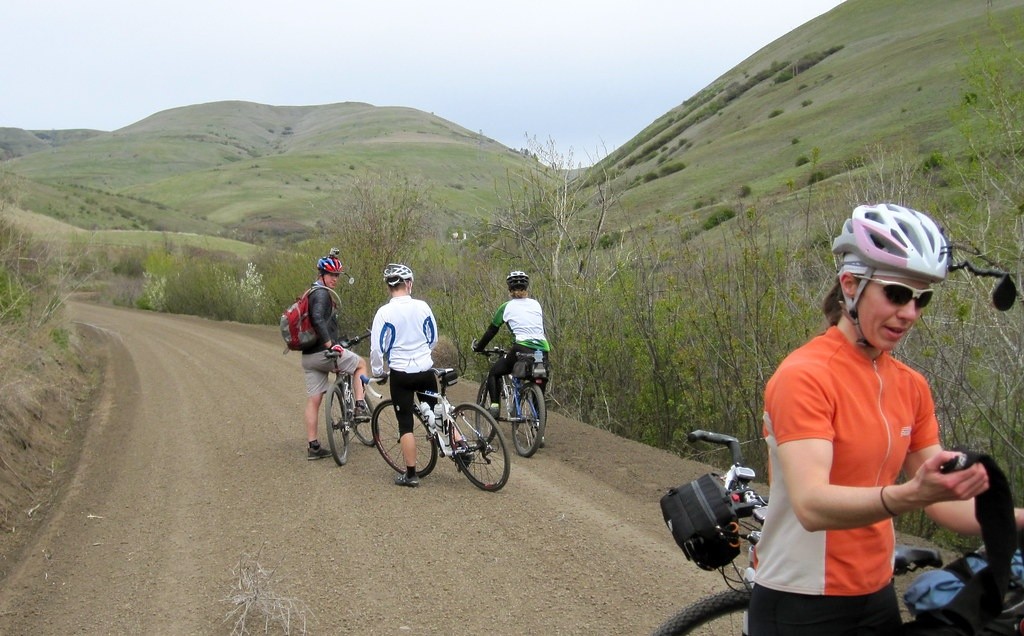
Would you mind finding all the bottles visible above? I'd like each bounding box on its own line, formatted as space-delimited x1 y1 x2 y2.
419 401 435 426
434 402 444 430
534 349 545 377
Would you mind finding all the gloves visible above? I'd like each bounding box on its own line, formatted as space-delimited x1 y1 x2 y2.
373 371 389 386
327 342 344 358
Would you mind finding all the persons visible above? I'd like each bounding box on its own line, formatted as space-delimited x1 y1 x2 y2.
370 263 469 487
474 270 551 448
748 203 1024 636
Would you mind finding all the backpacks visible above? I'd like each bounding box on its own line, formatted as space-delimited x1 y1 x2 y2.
278 285 336 356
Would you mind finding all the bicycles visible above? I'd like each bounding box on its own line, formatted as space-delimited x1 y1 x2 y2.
650 429 943 636
470 338 548 457
371 369 510 492
324 328 379 467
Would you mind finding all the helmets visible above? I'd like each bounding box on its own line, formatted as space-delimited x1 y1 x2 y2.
831 204 949 284
316 256 344 273
505 270 530 289
383 263 415 286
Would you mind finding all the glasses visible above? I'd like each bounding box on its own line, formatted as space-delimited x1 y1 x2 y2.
853 275 933 308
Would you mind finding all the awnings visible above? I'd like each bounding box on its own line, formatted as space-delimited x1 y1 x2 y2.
300 256 370 460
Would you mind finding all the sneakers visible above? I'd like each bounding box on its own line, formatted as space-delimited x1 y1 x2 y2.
457 446 474 472
394 473 420 487
539 435 545 449
354 403 372 420
307 445 333 460
486 408 499 419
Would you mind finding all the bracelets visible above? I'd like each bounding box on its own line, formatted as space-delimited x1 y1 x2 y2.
880 486 898 517
